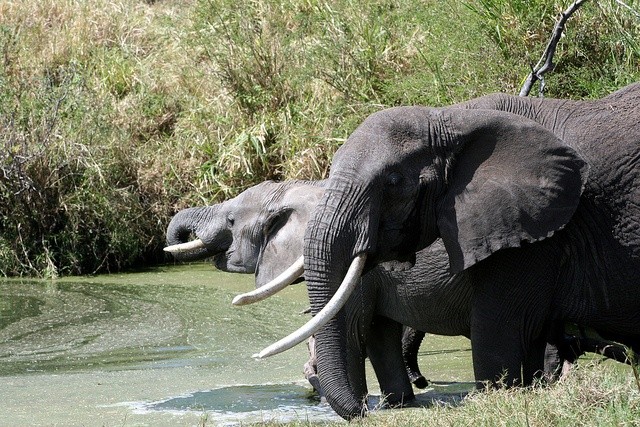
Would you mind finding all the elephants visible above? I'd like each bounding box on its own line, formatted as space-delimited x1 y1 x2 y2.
231 79 639 420
163 180 572 394
